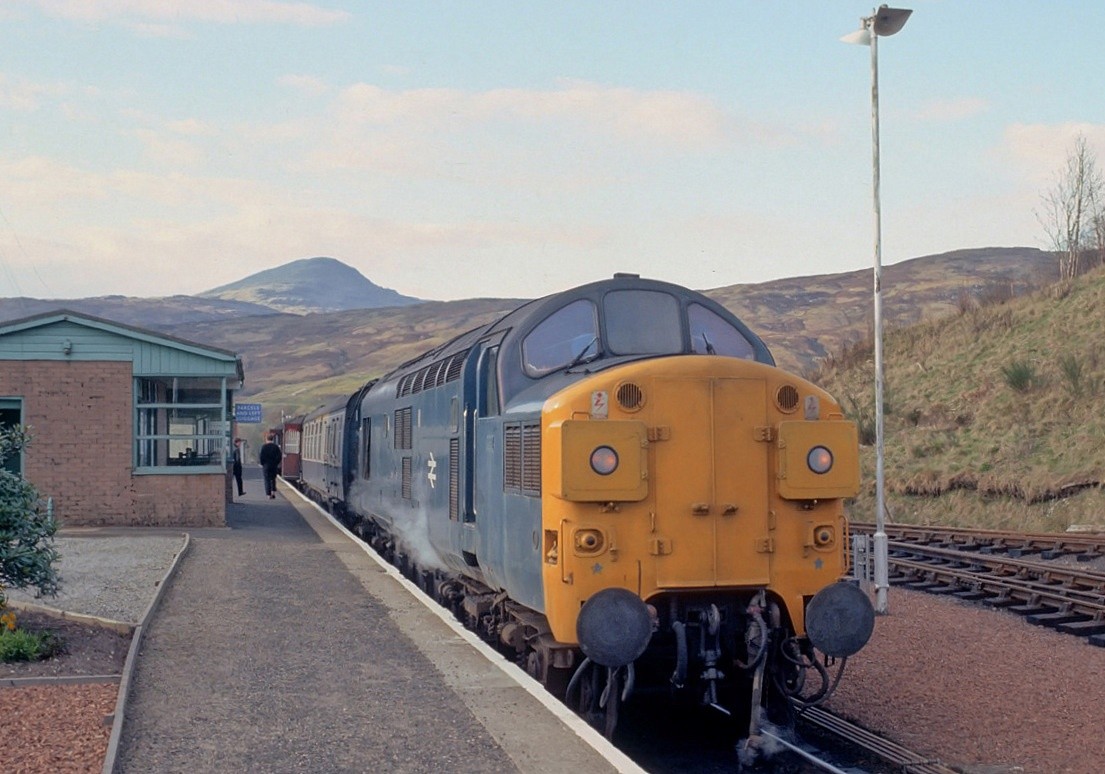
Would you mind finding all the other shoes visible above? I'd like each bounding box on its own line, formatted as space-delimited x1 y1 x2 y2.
239 492 246 496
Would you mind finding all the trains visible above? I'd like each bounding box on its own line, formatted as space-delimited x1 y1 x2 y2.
268 271 876 773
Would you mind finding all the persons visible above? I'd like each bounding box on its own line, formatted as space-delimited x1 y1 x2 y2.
227 438 247 497
260 434 282 500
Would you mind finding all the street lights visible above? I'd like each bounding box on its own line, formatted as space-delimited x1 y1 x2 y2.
839 3 913 613
